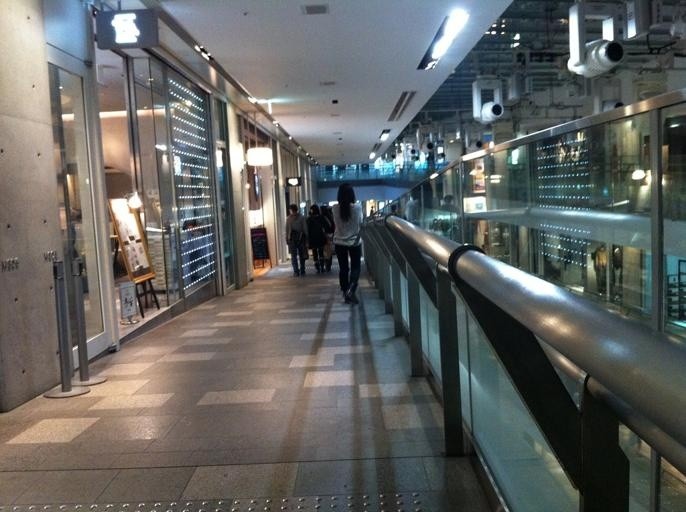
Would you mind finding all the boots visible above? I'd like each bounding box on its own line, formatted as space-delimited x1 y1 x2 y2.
343 281 359 304
315 258 332 273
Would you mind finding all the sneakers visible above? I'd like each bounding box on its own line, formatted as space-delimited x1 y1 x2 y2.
301 272 305 276
293 272 299 277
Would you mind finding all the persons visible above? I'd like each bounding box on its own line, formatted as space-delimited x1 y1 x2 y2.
331 181 363 304
501 226 509 258
387 189 460 241
284 203 336 277
612 246 623 294
595 245 607 295
